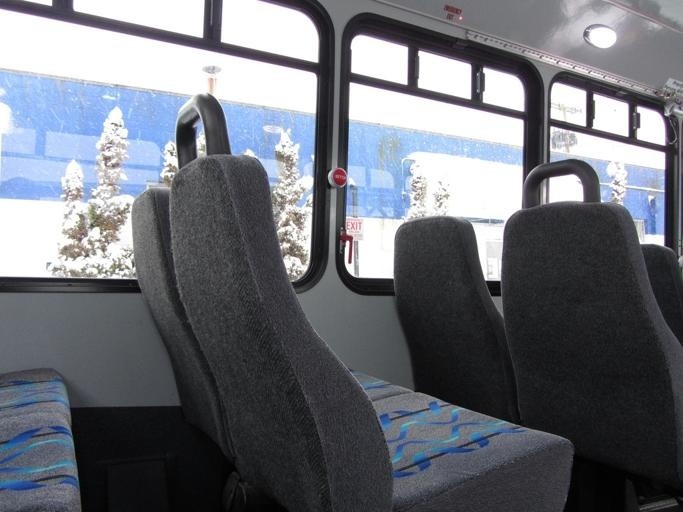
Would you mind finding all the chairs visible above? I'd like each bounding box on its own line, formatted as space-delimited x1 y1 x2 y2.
394 155 683 512
119 94 577 512
0 367 81 512
